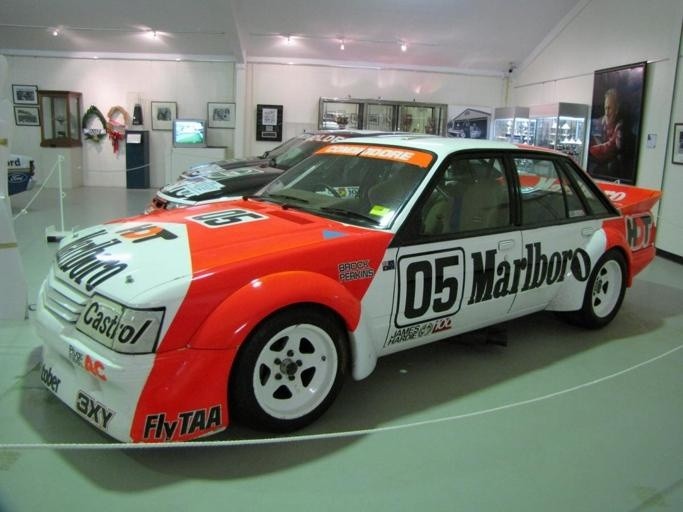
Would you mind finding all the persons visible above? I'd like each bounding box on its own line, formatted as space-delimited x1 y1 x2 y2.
587 87 623 179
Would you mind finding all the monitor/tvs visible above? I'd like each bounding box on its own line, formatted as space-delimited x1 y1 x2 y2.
171 118 208 148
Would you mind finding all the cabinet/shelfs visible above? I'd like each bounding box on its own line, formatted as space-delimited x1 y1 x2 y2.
36 90 84 148
531 103 589 170
494 107 529 144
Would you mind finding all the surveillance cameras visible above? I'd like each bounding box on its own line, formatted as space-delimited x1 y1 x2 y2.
507 64 515 74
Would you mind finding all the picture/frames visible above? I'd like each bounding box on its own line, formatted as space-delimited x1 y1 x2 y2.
671 122 683 165
206 101 235 130
586 61 648 185
13 106 41 126
11 84 38 105
150 101 177 132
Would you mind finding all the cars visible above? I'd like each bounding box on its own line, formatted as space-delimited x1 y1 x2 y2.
2 153 37 195
182 128 321 182
143 127 425 218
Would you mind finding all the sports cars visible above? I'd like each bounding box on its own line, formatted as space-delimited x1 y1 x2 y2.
33 126 663 451
